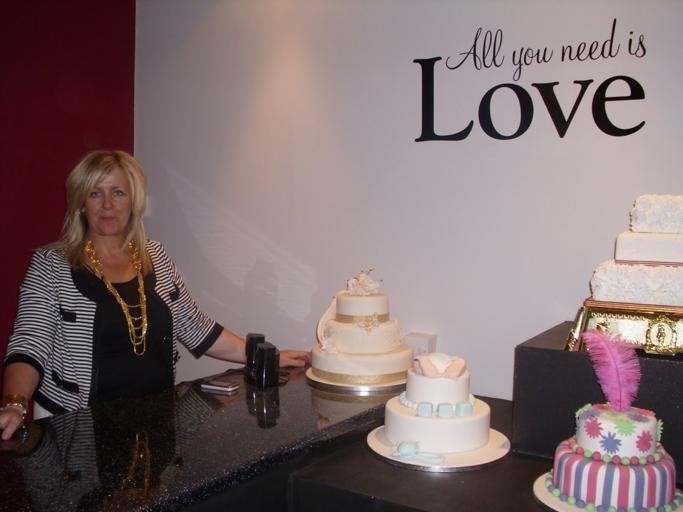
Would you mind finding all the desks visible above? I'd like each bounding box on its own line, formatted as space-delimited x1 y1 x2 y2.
285 394 553 511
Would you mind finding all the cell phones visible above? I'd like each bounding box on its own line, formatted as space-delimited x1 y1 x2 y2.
200 389 239 396
201 382 239 391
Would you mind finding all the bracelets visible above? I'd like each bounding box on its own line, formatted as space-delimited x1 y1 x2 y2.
0 393 27 420
17 424 28 448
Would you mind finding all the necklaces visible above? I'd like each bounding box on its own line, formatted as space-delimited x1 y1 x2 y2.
103 430 154 511
84 234 149 355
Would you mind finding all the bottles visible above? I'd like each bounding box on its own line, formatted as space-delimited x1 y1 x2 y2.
245 332 280 388
246 388 281 431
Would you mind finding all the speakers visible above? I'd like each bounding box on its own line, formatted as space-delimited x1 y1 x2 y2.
509 322 681 469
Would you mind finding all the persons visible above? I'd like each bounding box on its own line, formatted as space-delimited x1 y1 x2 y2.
17 373 236 511
1 149 313 441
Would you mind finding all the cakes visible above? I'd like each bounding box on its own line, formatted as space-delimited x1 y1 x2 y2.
545 402 683 512
312 289 414 384
591 193 683 306
385 355 491 454
